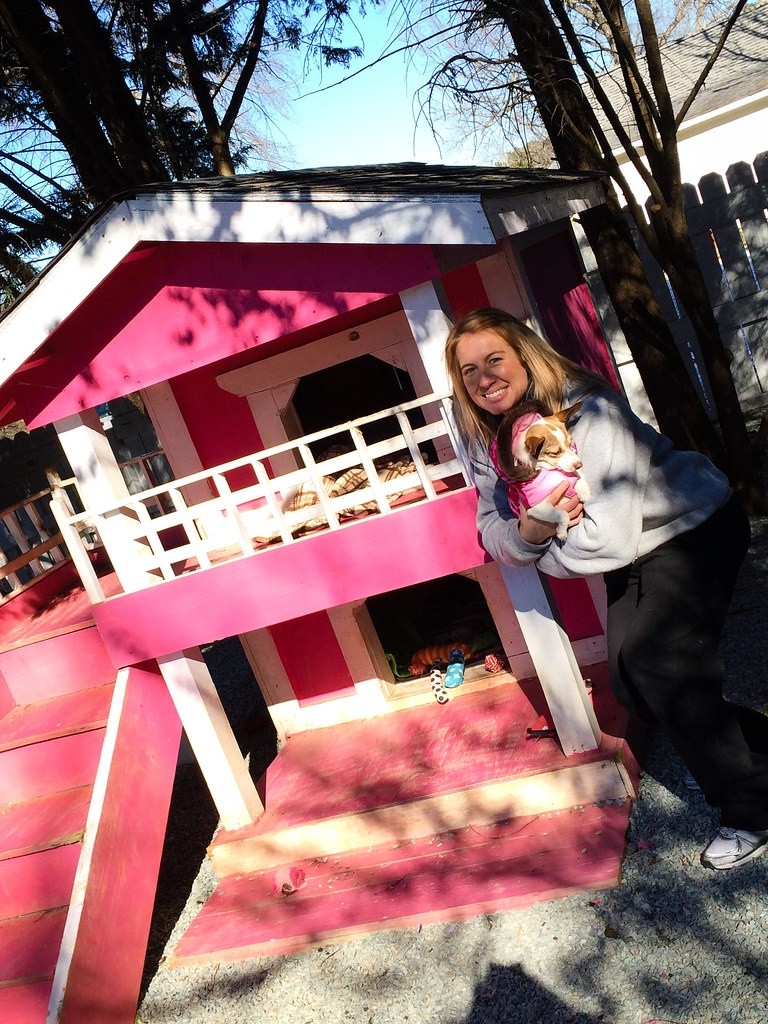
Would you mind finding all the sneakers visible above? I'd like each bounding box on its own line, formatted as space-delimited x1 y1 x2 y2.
685 763 700 789
700 826 768 869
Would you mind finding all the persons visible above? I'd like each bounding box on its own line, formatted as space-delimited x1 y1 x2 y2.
445 305 768 871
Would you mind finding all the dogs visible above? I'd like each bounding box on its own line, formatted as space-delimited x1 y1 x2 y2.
489 400 592 541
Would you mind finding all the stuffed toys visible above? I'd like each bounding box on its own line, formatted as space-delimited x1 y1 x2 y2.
385 639 479 705
